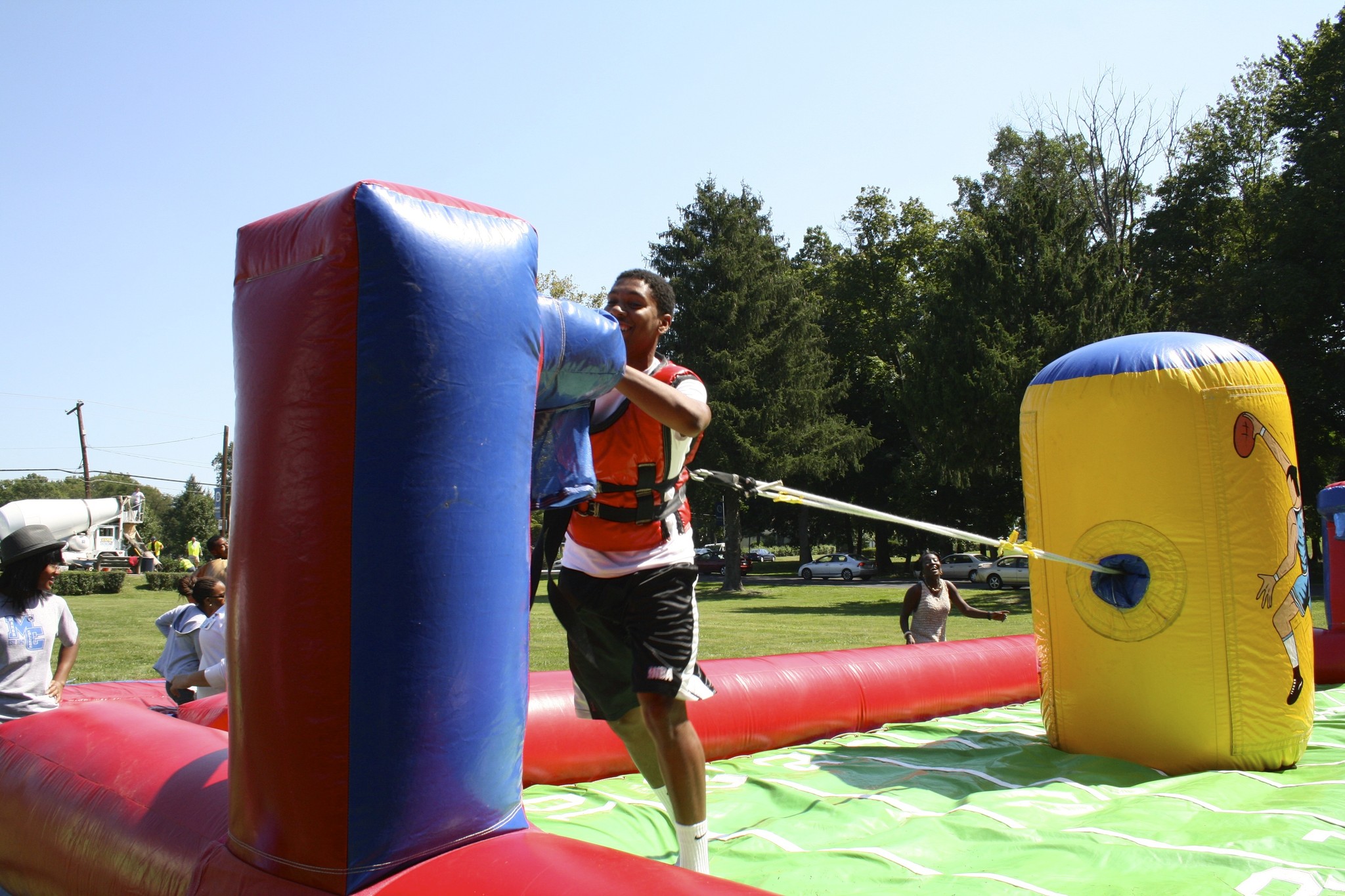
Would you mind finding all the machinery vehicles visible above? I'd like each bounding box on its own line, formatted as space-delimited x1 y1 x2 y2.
0 496 146 575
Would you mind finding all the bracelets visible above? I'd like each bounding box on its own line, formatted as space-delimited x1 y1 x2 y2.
987 611 992 620
904 631 912 639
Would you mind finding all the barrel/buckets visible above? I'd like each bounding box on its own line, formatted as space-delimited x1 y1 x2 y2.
141 557 154 572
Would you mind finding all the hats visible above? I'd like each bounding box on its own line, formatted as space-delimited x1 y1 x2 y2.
0 525 66 571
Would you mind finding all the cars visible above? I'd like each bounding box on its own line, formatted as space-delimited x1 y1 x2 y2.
798 553 878 582
977 555 1030 590
744 548 776 562
694 550 754 577
916 553 995 584
694 549 717 559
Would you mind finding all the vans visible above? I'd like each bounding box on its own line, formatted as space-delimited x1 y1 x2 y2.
702 543 725 551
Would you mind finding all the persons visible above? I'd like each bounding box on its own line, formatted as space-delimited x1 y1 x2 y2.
186 536 203 570
152 575 227 706
0 525 79 725
178 554 196 573
132 488 145 521
558 269 712 876
170 603 227 700
900 552 1010 644
189 535 229 586
148 538 164 561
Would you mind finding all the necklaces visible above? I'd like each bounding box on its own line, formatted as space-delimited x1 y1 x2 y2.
924 579 942 592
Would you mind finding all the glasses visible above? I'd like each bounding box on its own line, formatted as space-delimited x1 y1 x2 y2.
205 593 227 599
